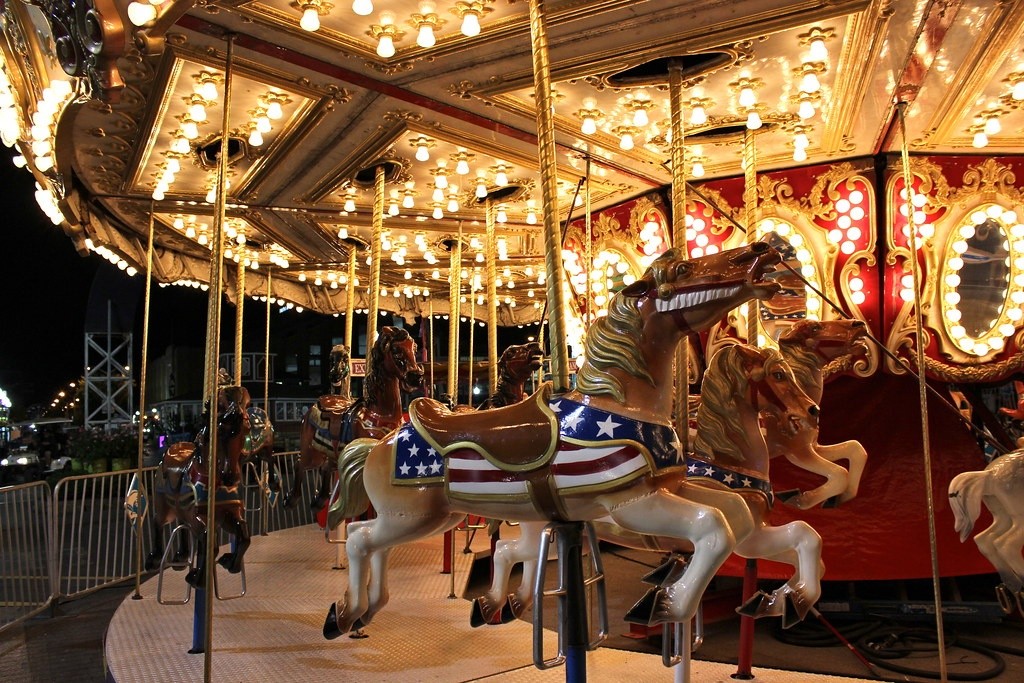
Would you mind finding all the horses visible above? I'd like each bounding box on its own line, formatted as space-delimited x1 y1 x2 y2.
145 368 279 590
283 242 1024 640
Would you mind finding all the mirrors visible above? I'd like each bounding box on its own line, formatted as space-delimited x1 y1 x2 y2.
754 231 809 341
956 216 1012 339
606 264 626 302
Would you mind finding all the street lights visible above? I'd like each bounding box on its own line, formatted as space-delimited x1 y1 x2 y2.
473 385 480 407
152 408 162 429
528 337 536 393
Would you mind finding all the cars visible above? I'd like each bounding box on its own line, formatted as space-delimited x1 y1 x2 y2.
1 448 40 471
